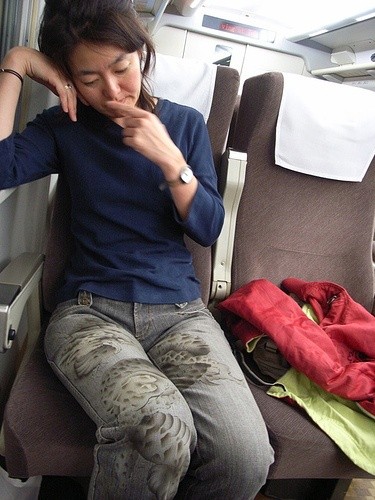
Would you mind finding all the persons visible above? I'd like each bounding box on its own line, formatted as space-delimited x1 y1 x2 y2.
0 0 275 500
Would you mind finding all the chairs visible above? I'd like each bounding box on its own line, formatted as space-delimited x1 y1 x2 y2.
0 53 375 500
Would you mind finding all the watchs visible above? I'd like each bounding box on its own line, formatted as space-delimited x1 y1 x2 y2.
165 166 194 186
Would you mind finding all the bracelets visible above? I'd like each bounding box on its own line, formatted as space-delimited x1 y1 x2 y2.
0 69 23 84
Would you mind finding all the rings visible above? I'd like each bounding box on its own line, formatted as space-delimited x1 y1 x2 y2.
65 84 73 89
